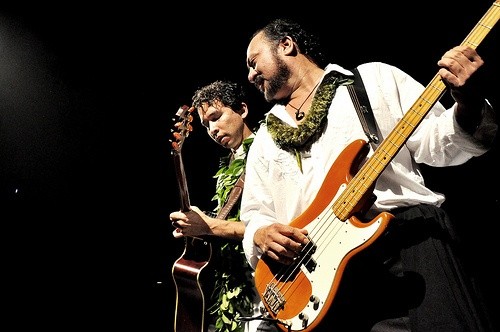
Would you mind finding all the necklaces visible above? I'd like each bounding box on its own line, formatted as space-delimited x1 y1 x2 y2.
287 82 319 120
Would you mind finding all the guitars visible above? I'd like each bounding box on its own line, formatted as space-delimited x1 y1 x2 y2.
254 0 500 332
168 104 216 332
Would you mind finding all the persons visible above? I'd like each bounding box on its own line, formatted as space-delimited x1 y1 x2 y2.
169 82 279 332
240 18 500 332
